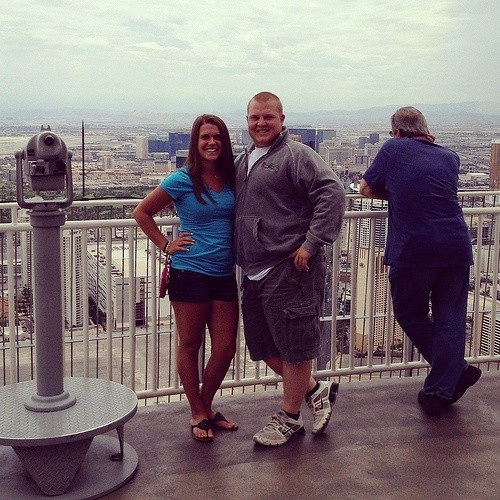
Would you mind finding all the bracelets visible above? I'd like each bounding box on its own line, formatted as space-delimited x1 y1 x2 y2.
163 241 169 253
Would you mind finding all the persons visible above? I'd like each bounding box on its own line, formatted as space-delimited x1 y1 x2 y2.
133 114 239 442
234 92 345 447
360 106 482 416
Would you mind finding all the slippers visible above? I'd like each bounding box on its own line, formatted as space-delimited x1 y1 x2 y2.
211 412 238 431
192 419 214 441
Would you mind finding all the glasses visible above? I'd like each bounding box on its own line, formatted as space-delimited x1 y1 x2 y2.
389 132 397 136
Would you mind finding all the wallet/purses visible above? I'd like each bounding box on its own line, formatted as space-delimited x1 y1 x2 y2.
160 268 167 298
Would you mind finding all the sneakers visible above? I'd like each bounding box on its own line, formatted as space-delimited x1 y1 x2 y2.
305 381 338 435
418 389 444 415
448 366 481 405
253 410 304 446
